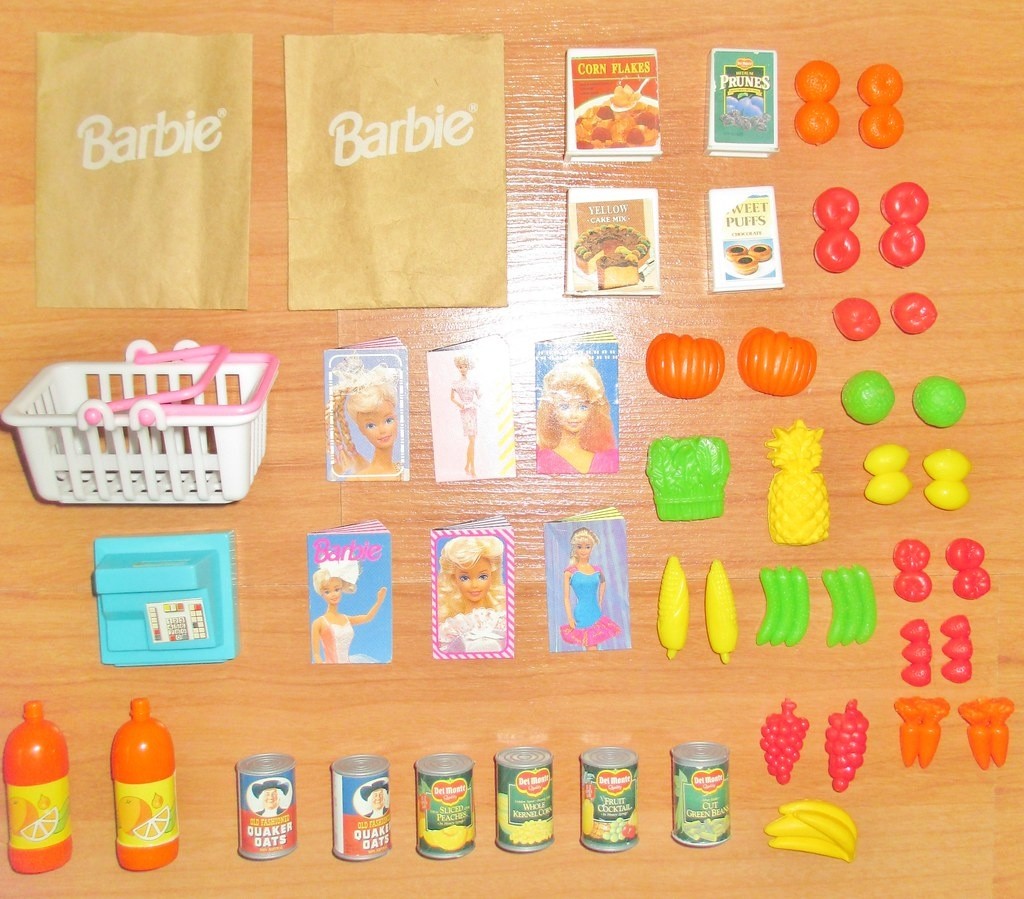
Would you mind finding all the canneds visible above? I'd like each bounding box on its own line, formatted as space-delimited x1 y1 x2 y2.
234 742 731 862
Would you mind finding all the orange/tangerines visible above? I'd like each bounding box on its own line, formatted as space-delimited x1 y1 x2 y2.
794 59 905 149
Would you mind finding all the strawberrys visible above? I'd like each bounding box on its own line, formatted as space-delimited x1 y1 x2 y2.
900 615 973 687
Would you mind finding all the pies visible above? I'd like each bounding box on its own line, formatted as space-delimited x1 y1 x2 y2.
726 244 773 277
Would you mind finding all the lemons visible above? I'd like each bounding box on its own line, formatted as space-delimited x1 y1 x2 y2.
841 370 972 511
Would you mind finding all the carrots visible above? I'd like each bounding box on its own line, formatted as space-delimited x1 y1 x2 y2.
895 697 1015 769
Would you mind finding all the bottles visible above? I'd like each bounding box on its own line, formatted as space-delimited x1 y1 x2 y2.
2 701 73 875
111 697 181 872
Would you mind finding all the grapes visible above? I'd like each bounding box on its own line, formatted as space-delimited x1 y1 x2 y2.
758 698 869 792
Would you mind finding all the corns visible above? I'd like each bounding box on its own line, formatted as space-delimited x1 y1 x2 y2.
656 557 740 665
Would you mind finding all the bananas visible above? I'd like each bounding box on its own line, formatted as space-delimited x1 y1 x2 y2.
765 800 857 862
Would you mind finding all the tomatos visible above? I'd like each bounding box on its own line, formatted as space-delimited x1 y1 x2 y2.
812 182 938 343
892 539 991 602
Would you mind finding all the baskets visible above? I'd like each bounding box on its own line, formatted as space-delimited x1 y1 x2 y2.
2 337 281 503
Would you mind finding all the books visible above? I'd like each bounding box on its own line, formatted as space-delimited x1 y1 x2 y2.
561 45 662 163
540 506 634 651
707 187 786 293
323 337 408 483
429 514 515 660
704 47 779 158
534 327 620 476
563 186 661 299
306 520 395 665
427 334 517 483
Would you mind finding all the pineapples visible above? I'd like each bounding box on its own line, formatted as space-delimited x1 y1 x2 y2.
764 420 832 545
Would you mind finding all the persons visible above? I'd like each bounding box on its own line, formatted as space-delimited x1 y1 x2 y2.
252 780 289 816
360 780 389 819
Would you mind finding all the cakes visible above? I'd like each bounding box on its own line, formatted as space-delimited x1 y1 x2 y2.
573 220 652 292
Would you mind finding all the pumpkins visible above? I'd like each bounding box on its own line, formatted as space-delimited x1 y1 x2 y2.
647 326 817 396
